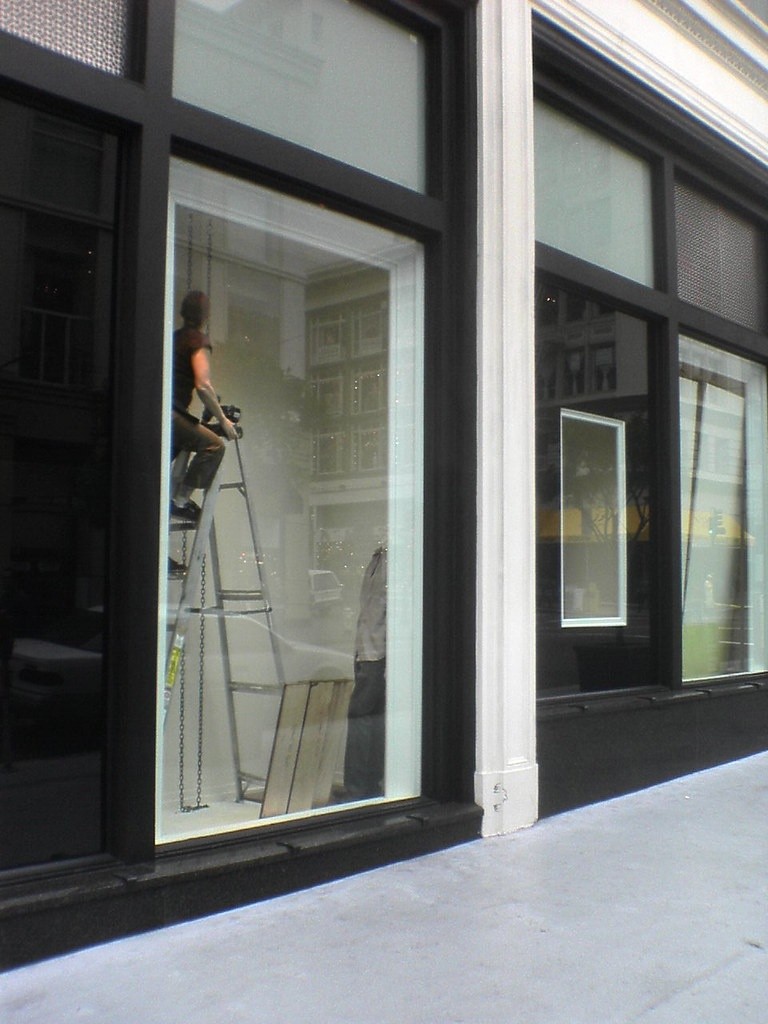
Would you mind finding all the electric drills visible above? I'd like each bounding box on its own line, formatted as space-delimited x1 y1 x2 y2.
200 396 241 425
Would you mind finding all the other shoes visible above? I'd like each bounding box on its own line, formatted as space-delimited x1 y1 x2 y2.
167 557 186 573
169 497 202 520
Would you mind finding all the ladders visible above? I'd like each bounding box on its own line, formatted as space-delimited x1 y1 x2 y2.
164 425 285 802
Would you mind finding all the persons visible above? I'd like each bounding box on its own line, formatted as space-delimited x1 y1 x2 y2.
168 290 240 572
333 547 386 798
